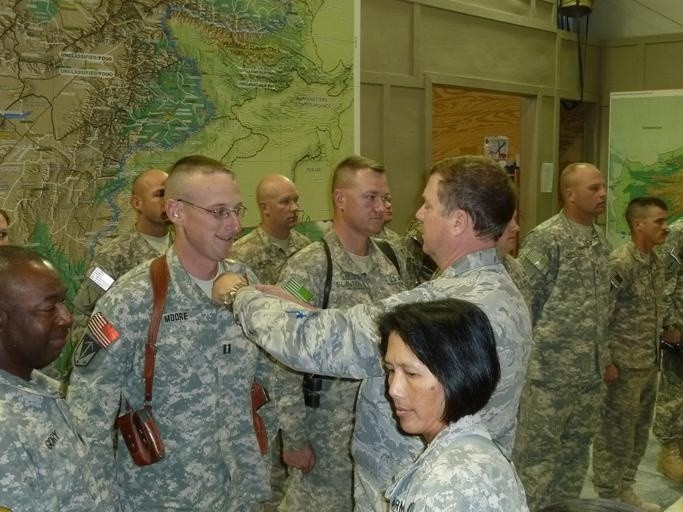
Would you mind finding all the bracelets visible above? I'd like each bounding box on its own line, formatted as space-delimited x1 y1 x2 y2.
223 280 245 308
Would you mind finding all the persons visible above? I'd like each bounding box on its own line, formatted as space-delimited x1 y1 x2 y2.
0 208 11 246
375 168 411 294
403 216 441 290
212 154 532 512
376 298 530 512
231 172 312 512
651 216 682 487
67 155 270 512
517 161 609 511
497 203 532 310
70 169 174 348
0 246 111 512
590 195 667 512
272 154 411 512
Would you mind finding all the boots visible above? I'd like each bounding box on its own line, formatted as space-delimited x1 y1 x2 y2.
655 437 683 483
617 485 662 511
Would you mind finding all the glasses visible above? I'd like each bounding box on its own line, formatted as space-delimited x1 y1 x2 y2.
183 200 248 219
